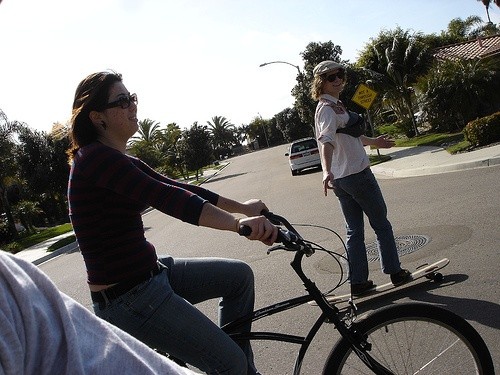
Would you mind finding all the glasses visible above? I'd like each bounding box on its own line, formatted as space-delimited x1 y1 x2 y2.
327 72 343 82
107 93 138 108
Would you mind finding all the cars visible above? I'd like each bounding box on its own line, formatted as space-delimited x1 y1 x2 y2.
285 137 322 176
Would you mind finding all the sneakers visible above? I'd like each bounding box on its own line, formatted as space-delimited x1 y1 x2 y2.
351 280 373 294
390 270 410 284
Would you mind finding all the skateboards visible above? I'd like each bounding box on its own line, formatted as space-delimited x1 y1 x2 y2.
308 258 452 312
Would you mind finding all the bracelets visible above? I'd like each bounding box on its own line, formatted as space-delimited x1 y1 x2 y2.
235 217 241 233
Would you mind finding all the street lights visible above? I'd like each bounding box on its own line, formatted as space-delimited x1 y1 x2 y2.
259 61 306 137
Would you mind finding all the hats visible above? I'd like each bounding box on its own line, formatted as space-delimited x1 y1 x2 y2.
313 61 343 77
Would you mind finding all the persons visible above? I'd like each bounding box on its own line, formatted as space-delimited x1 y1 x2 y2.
66 72 277 375
311 60 411 296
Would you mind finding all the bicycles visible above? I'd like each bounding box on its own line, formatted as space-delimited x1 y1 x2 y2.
149 208 495 375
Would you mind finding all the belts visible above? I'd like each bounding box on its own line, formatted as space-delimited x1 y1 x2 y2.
91 263 162 310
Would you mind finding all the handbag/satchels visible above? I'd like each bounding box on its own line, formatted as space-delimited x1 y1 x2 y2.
336 109 365 137
